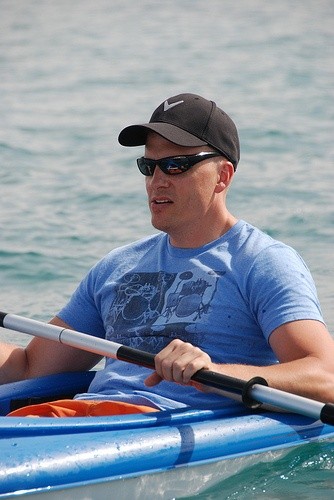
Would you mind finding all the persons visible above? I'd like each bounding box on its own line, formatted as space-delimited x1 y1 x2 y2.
0 94 334 408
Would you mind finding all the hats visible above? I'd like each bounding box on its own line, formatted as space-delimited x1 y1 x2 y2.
118 94 240 173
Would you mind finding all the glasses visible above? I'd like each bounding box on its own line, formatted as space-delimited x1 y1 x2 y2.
137 151 221 176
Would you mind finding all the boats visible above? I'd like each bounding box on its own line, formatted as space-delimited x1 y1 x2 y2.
0 365 334 500
